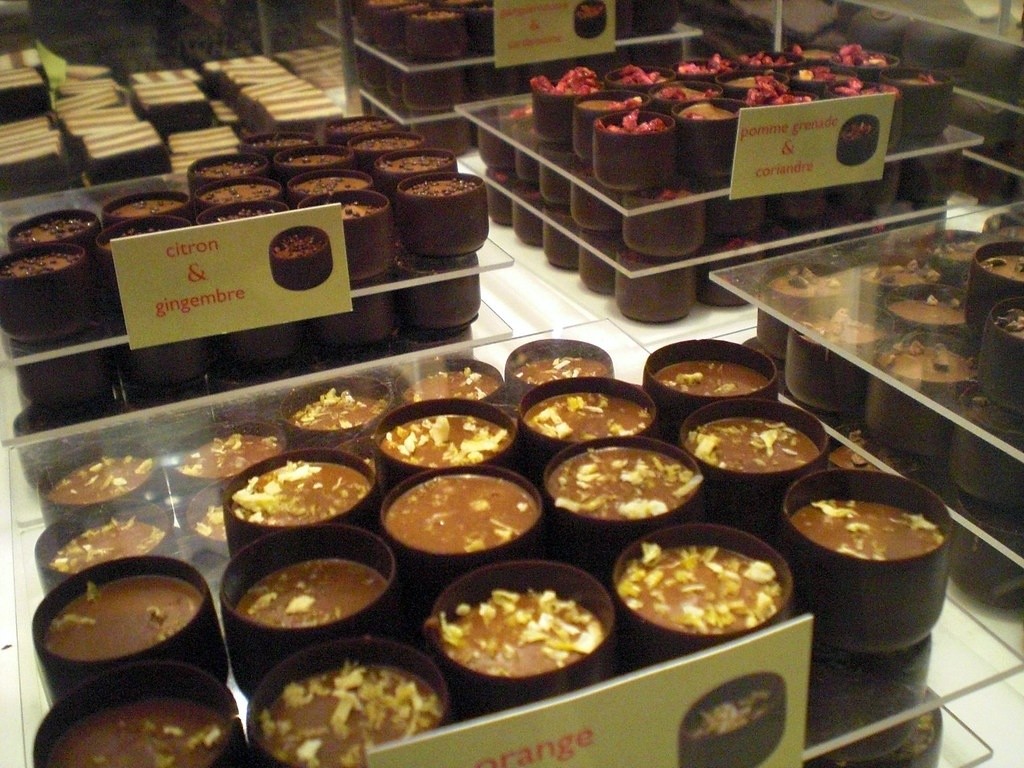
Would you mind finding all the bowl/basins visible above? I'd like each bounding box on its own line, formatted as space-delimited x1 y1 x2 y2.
573 0 608 38
0 1 1024 768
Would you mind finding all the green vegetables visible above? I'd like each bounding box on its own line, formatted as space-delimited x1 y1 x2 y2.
48 351 938 768
